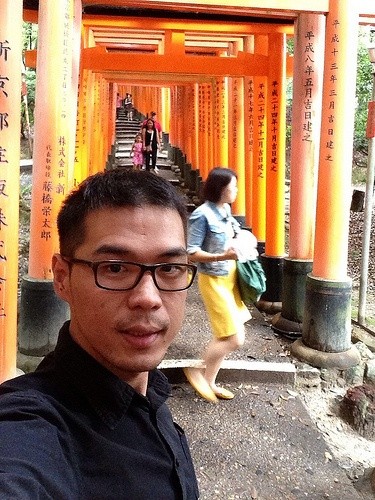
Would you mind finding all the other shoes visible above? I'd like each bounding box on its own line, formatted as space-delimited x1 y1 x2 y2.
142 165 146 170
154 165 160 174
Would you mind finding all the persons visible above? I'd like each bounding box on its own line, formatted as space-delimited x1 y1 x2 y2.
142 119 162 175
125 93 134 121
185 167 252 405
117 93 122 122
130 135 146 170
1 168 199 499
143 112 163 172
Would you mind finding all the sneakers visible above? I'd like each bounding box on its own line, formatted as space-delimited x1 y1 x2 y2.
184 368 216 402
195 387 234 399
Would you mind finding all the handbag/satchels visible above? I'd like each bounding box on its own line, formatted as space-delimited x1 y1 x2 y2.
236 260 266 302
146 145 152 152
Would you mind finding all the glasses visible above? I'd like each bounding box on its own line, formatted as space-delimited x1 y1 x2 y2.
59 254 197 292
